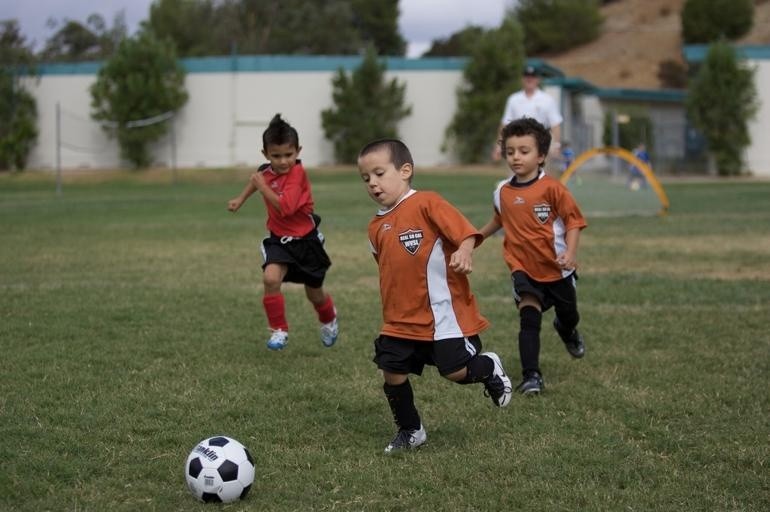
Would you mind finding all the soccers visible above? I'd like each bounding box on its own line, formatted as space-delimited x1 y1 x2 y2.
185 436 256 504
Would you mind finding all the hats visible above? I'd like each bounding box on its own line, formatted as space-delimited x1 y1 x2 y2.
523 66 539 76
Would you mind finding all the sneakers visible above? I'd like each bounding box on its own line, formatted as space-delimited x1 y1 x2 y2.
385 424 427 454
481 351 512 407
266 327 289 349
515 370 545 397
553 316 585 358
320 307 338 347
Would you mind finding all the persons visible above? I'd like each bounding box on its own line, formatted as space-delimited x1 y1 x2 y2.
624 143 654 192
492 64 566 161
558 142 579 188
477 115 588 398
227 112 341 350
356 139 514 454
